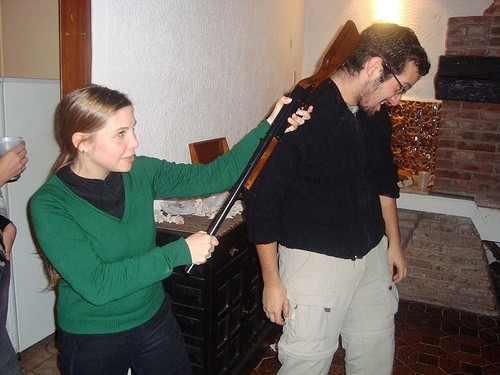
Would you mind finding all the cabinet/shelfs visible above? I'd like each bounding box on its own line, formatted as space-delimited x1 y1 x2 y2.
156 201 279 375
0 77 61 361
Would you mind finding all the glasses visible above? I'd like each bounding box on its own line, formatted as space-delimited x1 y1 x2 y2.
370 51 409 94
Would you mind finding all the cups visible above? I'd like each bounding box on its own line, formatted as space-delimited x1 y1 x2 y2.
418 171 430 191
0 136 23 181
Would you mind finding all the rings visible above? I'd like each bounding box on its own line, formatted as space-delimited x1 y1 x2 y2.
206 252 211 260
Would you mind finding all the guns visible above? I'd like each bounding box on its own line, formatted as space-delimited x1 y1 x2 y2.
185 20 361 275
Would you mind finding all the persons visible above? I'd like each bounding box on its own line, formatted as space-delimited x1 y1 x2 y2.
0 140 28 375
242 23 431 375
27 84 313 375
